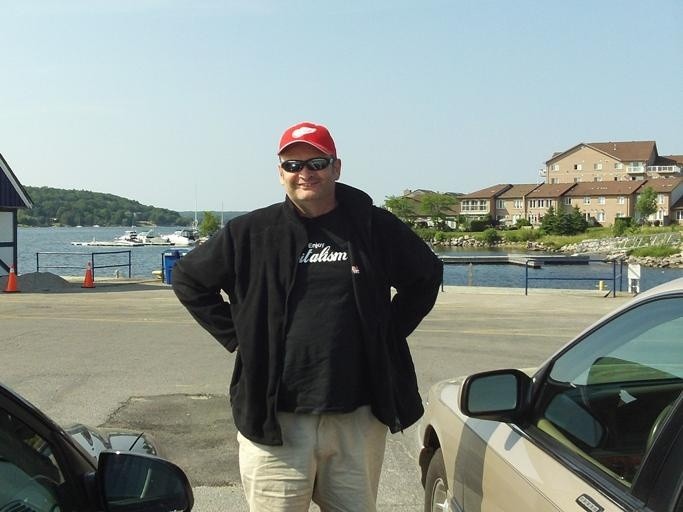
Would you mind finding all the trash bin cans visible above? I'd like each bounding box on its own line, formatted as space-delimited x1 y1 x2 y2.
164 250 189 284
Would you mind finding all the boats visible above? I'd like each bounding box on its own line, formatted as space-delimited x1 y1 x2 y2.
69 185 227 247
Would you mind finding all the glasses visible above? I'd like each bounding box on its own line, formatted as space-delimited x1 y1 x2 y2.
279 155 336 173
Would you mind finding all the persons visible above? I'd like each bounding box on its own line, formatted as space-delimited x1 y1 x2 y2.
172 122 441 512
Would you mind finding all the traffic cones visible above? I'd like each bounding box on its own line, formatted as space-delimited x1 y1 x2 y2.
80 262 99 290
2 264 24 295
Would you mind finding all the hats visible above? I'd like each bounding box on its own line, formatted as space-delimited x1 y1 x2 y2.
277 122 338 161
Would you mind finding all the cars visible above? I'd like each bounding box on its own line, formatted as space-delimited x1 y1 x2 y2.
415 276 683 512
1 380 195 512
